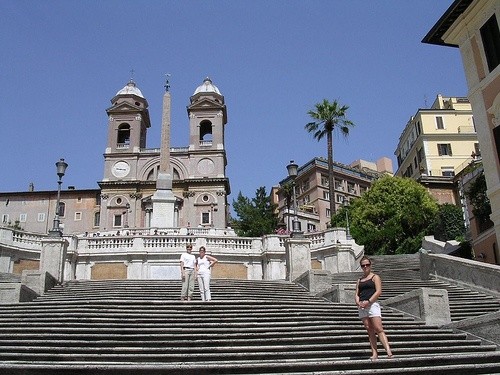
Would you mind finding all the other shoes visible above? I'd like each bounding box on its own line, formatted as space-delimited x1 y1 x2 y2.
387 354 393 357
370 356 378 359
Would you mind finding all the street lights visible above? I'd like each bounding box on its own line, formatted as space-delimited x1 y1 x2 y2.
285 159 304 240
48 158 70 239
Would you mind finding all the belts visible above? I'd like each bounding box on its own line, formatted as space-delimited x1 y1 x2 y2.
184 267 190 269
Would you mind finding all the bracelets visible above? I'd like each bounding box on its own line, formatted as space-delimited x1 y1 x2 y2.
368 300 371 304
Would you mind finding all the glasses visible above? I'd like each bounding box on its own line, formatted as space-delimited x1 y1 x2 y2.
361 264 370 268
186 246 191 248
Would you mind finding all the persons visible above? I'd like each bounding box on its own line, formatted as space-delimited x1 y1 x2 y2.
83 230 194 247
355 257 394 359
196 246 217 301
180 245 196 301
